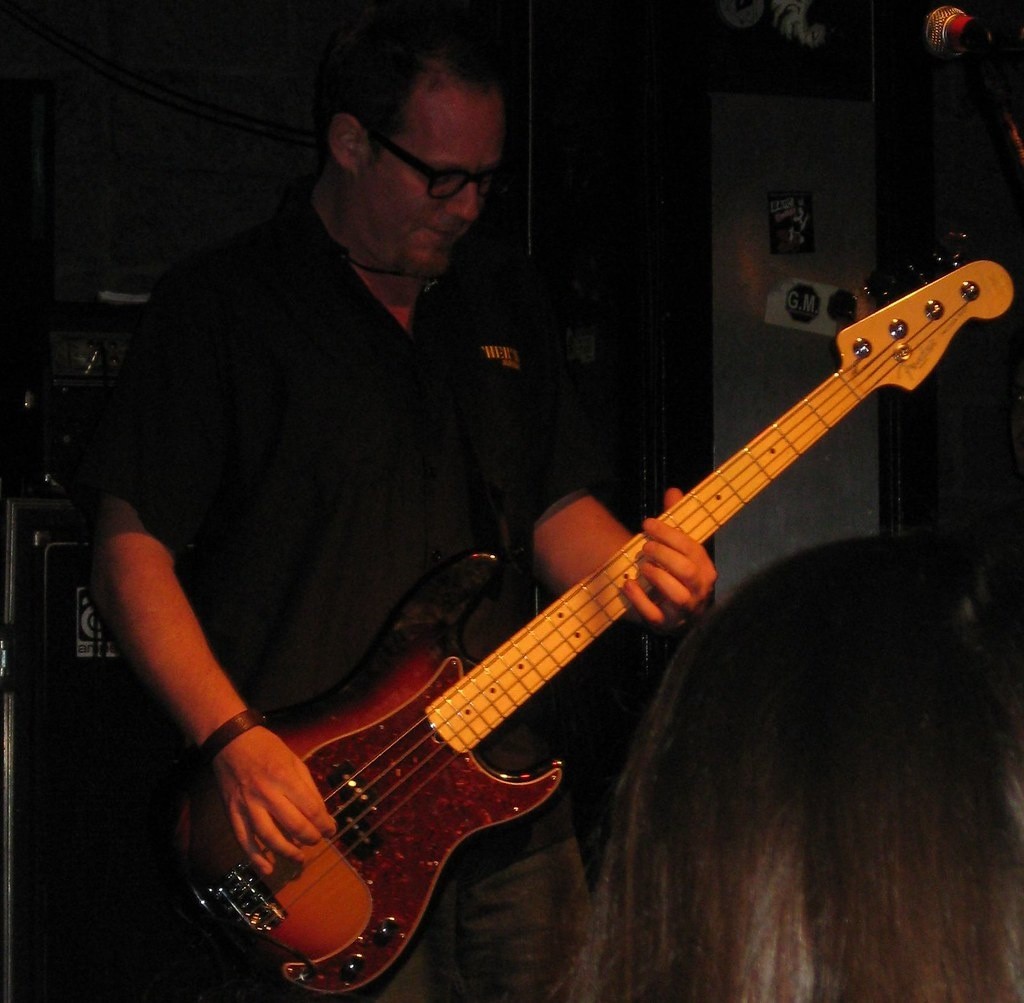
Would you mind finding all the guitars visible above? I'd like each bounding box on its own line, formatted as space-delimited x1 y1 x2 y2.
176 220 1015 995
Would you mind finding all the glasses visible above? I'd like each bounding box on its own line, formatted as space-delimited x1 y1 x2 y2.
368 132 523 199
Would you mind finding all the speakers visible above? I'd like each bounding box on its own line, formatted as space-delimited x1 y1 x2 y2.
0 495 227 1003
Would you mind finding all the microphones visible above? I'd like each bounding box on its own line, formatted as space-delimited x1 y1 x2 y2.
924 5 993 60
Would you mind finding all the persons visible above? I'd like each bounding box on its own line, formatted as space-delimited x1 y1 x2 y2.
86 14 722 1003
554 521 1023 1003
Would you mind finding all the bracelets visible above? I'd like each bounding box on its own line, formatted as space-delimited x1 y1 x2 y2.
197 708 265 763
